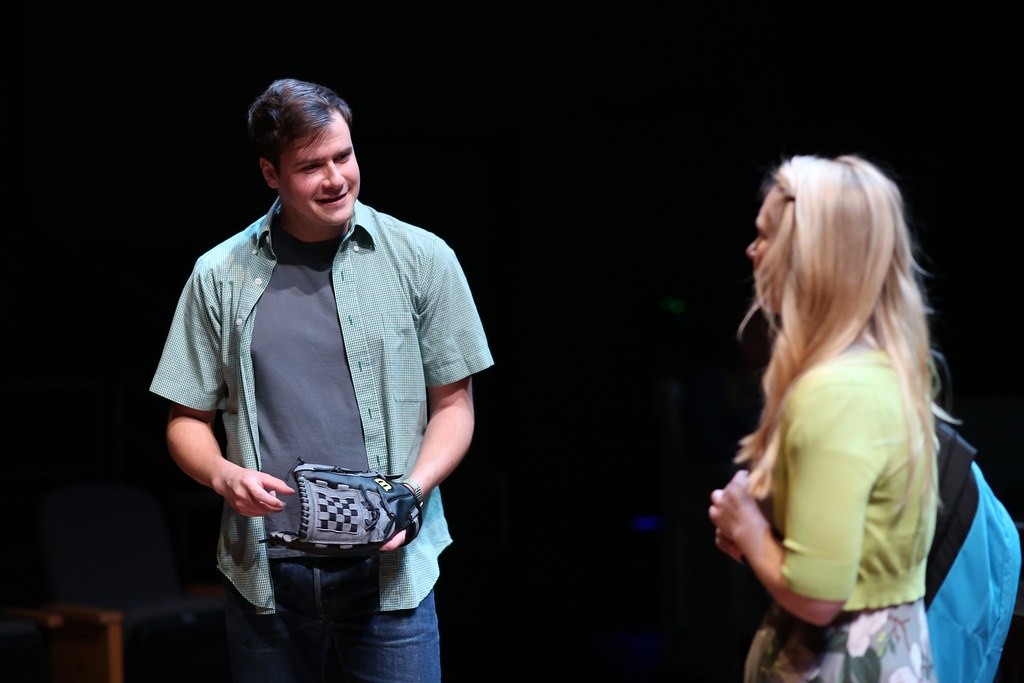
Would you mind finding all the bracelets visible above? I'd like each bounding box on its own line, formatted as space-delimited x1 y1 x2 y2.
403 479 424 509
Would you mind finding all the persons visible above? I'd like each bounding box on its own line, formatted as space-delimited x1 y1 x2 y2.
708 156 938 683
150 79 493 683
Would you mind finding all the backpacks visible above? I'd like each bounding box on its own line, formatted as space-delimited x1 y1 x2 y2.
926 404 1022 683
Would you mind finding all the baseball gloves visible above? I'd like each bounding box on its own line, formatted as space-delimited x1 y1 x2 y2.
268 463 422 554
